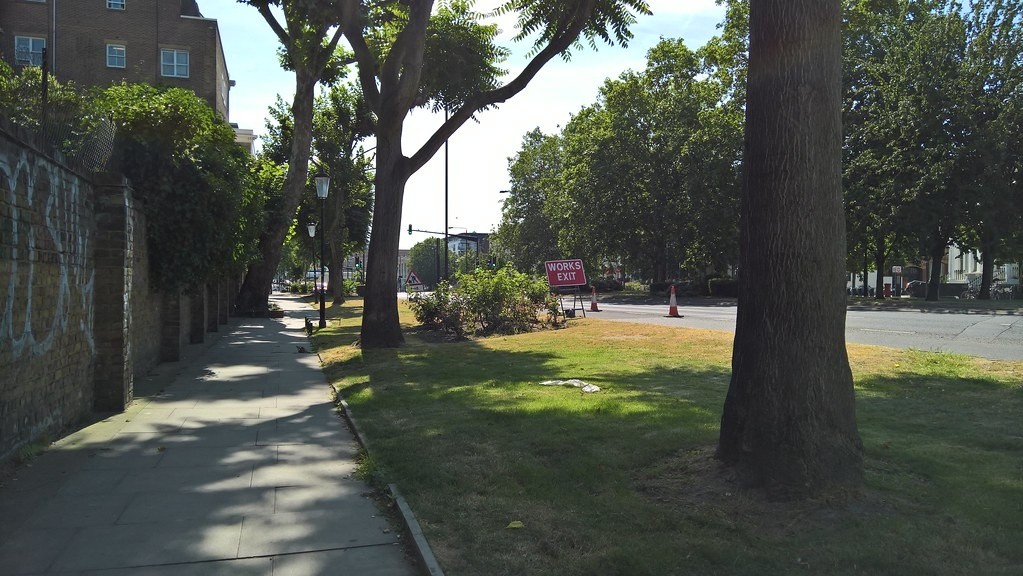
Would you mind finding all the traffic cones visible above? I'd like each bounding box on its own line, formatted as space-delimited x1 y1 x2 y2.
586 285 603 312
663 285 684 317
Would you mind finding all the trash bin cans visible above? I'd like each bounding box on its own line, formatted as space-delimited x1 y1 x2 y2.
884 283 891 297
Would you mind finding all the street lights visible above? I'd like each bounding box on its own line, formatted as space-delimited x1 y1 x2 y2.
314 164 332 327
306 220 318 304
448 227 467 291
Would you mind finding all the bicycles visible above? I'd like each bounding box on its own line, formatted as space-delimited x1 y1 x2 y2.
960 284 981 300
990 278 1002 301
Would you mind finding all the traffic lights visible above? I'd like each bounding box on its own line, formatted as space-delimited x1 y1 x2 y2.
408 224 412 235
354 256 359 268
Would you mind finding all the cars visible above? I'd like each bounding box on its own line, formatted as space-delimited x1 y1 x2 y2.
314 283 327 293
904 280 925 297
272 285 287 292
